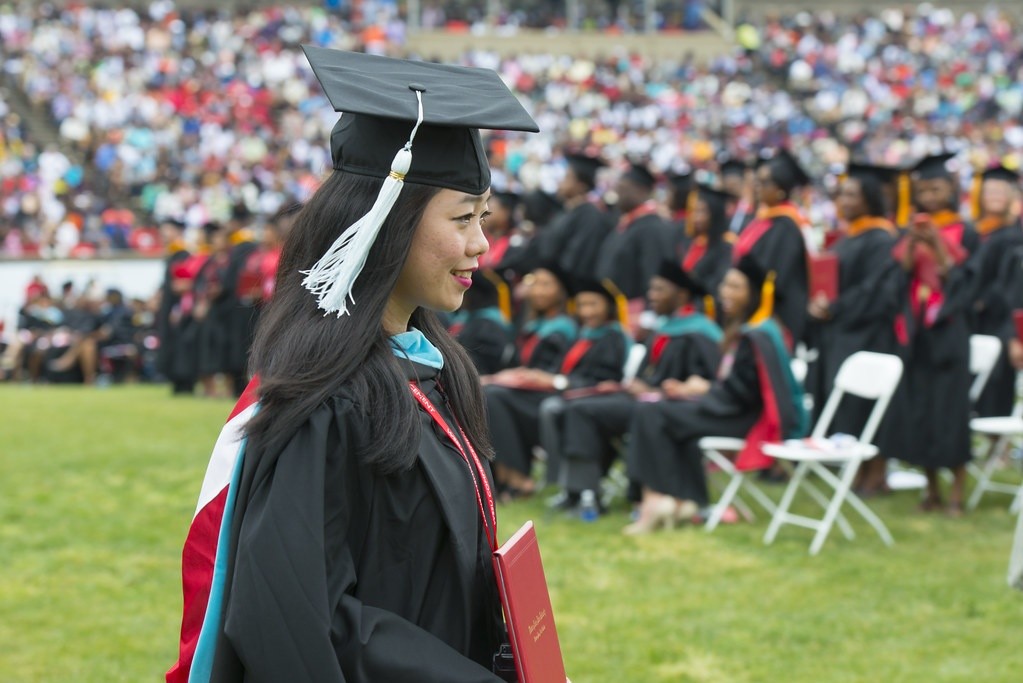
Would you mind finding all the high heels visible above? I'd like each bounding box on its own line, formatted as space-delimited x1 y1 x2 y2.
675 499 698 524
623 495 677 535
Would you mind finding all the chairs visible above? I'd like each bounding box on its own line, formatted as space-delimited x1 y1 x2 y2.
698 348 904 557
884 333 1023 512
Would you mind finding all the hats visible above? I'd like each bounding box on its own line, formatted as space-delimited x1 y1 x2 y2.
733 256 783 330
847 164 911 228
202 221 224 233
560 140 754 224
472 270 510 322
539 250 572 290
570 276 632 330
910 150 962 214
297 44 540 317
233 205 256 221
649 258 715 319
768 144 815 209
971 164 1020 221
159 217 190 228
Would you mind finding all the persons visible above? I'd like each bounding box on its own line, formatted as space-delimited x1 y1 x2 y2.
0 0 1022 534
162 42 539 682
